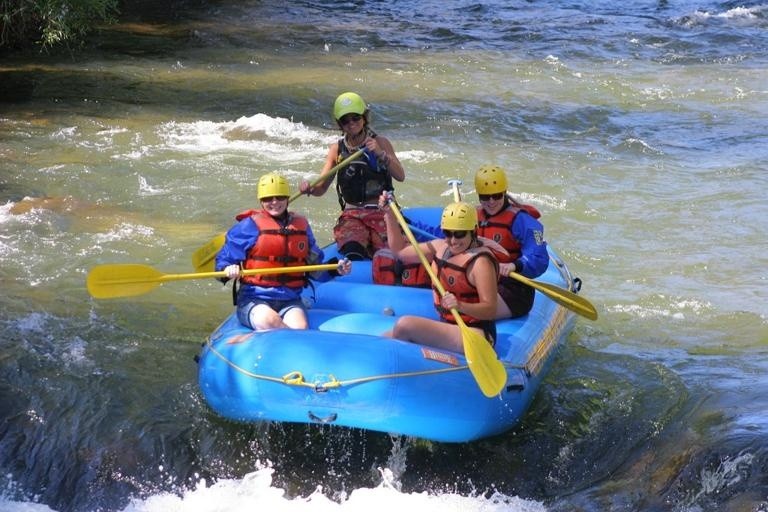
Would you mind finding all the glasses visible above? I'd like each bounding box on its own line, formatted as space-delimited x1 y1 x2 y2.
442 230 467 239
340 114 361 125
479 193 504 201
262 197 286 201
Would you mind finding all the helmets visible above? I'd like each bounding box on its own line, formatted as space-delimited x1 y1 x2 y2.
257 173 290 198
438 203 479 230
334 91 367 119
475 164 509 194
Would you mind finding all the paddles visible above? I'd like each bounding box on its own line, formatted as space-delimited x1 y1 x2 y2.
191 144 369 279
490 179 598 322
382 190 508 398
86 256 349 301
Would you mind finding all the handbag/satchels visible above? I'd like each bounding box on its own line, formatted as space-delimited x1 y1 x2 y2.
371 244 433 288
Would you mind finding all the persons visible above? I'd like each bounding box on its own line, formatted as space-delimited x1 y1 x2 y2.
299 91 411 261
215 173 352 331
378 190 500 355
474 165 549 321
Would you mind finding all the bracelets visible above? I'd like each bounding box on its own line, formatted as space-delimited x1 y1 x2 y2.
378 147 391 168
